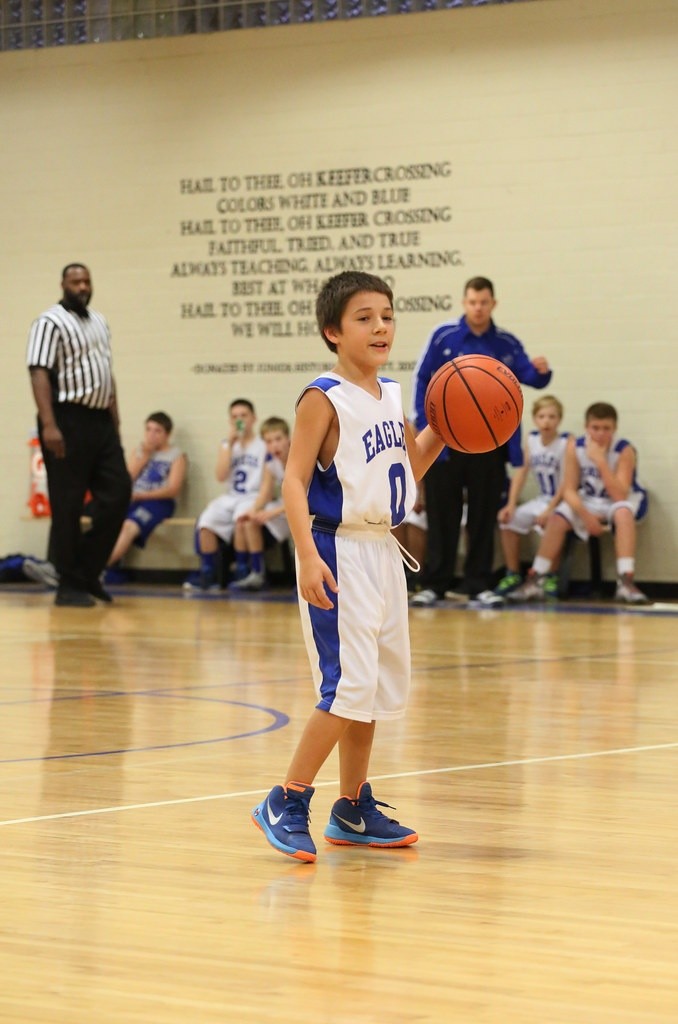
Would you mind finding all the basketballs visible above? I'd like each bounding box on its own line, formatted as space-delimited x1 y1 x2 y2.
422 352 526 455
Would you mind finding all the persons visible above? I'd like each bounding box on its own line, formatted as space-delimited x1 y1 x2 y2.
506 402 650 605
413 276 552 609
26 263 133 607
494 395 574 598
251 270 445 864
391 411 511 598
229 416 291 593
182 398 266 595
80 411 189 598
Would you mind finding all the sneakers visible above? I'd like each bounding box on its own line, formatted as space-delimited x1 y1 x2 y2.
325 782 418 847
182 571 235 593
494 571 522 595
250 781 317 861
466 591 505 608
613 573 649 603
23 557 60 588
543 572 557 602
229 571 273 590
410 590 447 606
506 569 547 602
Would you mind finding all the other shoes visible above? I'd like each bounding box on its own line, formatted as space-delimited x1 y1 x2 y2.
87 578 113 603
56 580 96 607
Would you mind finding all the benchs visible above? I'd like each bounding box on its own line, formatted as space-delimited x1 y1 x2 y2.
81 516 612 598
